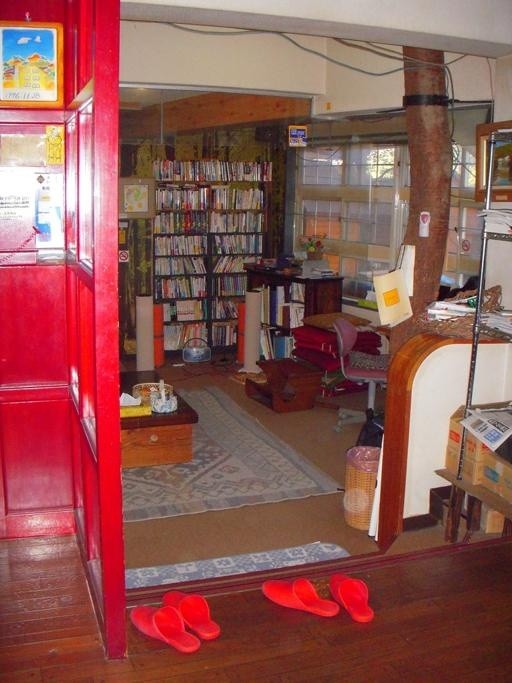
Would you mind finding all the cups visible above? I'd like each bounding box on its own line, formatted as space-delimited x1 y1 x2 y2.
454 271 465 289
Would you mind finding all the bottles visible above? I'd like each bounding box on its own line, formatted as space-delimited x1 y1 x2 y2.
151 389 177 412
255 256 262 264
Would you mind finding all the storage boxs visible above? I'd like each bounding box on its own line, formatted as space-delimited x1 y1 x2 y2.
445 401 511 506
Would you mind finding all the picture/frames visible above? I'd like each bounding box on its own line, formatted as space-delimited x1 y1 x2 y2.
2 18 66 111
474 120 512 204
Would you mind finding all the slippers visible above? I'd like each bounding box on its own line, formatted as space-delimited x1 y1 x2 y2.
130 589 221 655
261 572 375 624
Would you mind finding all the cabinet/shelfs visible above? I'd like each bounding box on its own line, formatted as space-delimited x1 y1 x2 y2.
246 269 344 415
145 141 277 363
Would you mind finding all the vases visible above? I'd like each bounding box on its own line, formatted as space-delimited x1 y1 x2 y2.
306 251 324 258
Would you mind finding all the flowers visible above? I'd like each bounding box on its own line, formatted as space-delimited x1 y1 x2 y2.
304 234 330 250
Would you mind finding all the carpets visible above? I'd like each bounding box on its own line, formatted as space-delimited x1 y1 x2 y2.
119 384 343 522
126 538 350 590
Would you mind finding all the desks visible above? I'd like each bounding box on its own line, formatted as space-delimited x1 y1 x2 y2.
430 467 512 547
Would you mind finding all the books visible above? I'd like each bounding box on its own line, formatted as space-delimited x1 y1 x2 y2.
400 244 416 297
474 207 511 235
477 308 511 336
370 267 413 329
426 294 478 321
393 243 412 272
153 158 275 352
248 276 305 360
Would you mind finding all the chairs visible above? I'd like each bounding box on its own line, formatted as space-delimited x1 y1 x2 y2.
330 313 394 433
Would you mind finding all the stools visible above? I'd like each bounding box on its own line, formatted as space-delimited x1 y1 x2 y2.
244 356 325 414
